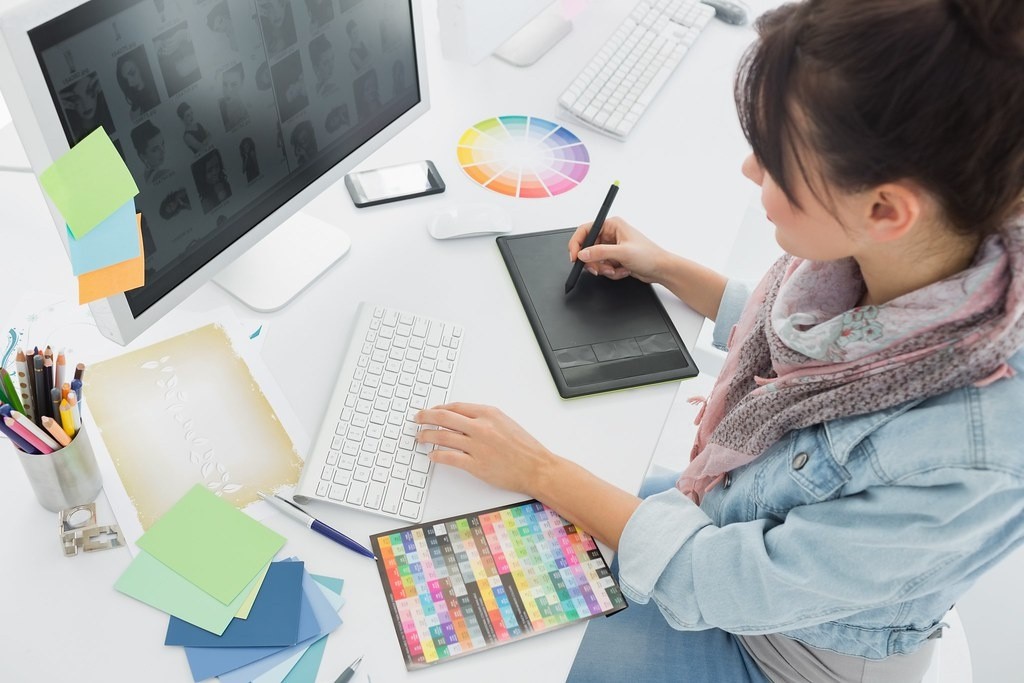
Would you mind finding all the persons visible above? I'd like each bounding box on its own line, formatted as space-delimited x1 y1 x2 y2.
415 0 1024 683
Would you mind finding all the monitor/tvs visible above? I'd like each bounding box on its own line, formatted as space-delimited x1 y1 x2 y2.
435 0 571 67
0 0 432 344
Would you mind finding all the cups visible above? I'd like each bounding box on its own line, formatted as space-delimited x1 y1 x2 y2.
15 417 104 513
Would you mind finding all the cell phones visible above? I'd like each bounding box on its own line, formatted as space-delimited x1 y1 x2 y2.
344 159 446 208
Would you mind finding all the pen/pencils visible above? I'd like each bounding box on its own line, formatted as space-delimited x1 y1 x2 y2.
562 167 624 299
0 339 89 463
249 484 382 567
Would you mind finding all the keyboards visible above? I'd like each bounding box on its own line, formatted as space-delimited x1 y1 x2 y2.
552 0 717 141
292 302 465 527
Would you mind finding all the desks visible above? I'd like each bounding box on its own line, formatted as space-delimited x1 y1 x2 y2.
0 0 785 683
